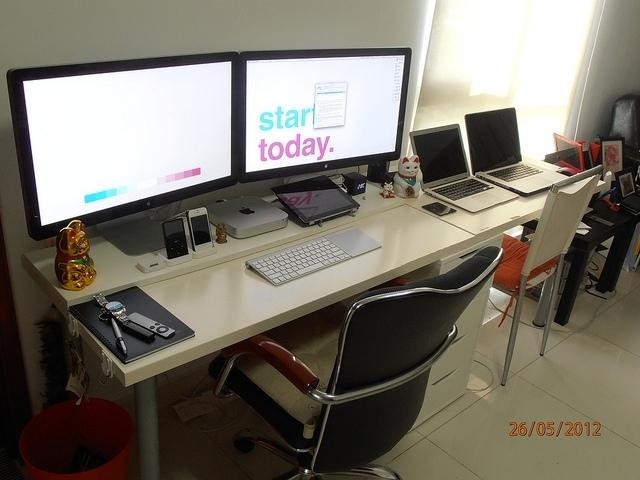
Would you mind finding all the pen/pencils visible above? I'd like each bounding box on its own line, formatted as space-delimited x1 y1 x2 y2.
110 317 129 358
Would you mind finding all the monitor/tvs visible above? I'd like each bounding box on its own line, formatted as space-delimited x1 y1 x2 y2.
6 52 239 255
239 47 412 185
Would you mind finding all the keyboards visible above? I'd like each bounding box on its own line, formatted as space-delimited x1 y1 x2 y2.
245 226 382 286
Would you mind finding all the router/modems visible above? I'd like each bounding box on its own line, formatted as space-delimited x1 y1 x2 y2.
205 196 288 239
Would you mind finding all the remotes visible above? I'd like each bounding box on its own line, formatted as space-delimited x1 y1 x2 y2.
121 322 155 343
128 313 176 338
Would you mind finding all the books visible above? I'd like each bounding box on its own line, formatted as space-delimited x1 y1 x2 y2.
621 207 640 216
622 193 640 212
68 284 196 365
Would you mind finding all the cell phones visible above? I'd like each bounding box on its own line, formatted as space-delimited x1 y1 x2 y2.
187 206 214 253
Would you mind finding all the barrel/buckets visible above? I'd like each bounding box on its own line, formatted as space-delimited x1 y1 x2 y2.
20 399 134 480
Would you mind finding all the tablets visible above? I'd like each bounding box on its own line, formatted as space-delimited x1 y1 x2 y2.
269 175 360 223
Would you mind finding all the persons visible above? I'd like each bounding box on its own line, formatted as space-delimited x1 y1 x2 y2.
606 146 618 166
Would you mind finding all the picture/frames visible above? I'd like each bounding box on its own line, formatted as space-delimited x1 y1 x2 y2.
600 137 624 174
615 167 636 200
553 133 585 173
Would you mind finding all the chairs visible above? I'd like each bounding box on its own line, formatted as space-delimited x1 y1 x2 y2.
492 164 604 386
209 246 504 480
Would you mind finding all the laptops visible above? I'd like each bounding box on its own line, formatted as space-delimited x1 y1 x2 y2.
409 124 519 213
464 107 570 197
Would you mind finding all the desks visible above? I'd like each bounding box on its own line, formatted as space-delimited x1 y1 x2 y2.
520 192 640 326
21 155 606 480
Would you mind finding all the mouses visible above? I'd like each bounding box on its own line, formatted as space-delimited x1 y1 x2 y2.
556 167 577 177
